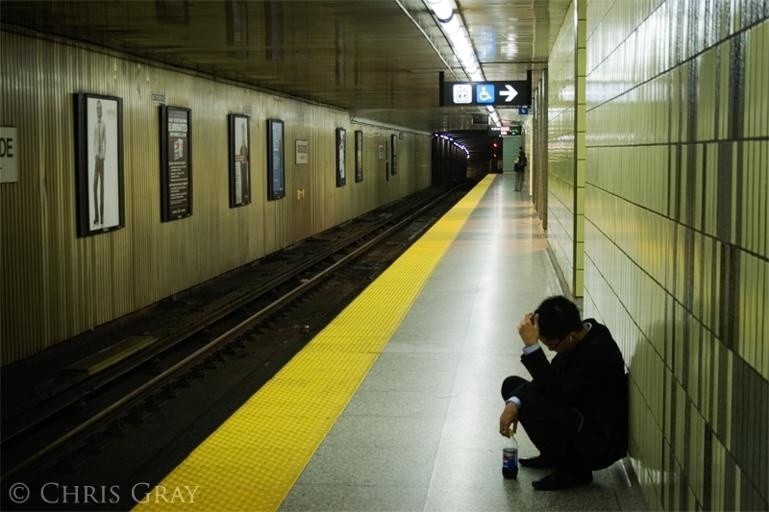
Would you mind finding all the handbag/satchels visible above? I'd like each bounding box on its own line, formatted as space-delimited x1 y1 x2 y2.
514 163 520 172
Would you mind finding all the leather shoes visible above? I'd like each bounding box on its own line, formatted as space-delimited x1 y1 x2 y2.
531 467 594 491
518 452 565 468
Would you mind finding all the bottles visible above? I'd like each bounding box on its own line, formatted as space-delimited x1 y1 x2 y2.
502 429 519 482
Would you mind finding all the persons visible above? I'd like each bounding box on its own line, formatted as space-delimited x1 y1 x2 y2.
238 122 248 202
497 296 629 492
91 99 108 225
338 130 345 179
513 152 527 192
